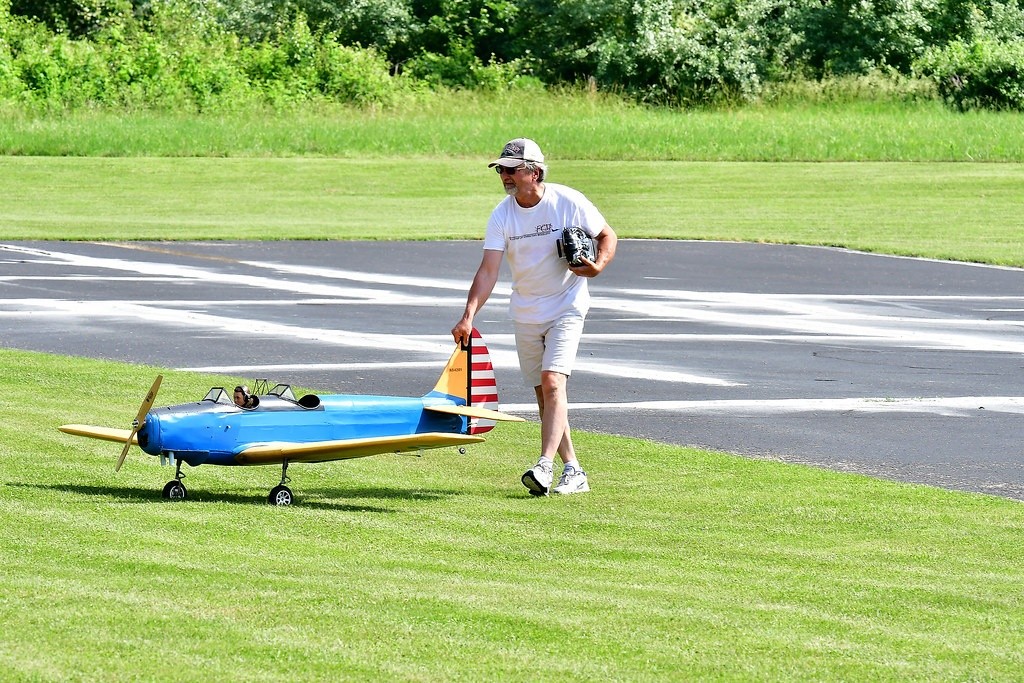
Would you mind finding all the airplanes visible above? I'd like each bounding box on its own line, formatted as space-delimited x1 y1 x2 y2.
55 324 527 507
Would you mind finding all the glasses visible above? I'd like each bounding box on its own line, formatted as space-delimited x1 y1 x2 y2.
495 166 526 175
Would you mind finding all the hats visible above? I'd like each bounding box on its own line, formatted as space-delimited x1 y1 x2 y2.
488 138 544 168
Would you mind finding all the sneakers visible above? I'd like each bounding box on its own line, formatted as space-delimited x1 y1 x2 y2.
553 466 589 494
521 461 558 498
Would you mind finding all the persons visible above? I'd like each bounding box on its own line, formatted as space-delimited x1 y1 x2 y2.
451 136 618 497
234 385 255 409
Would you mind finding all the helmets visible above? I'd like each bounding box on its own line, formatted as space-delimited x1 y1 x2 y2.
237 385 252 399
